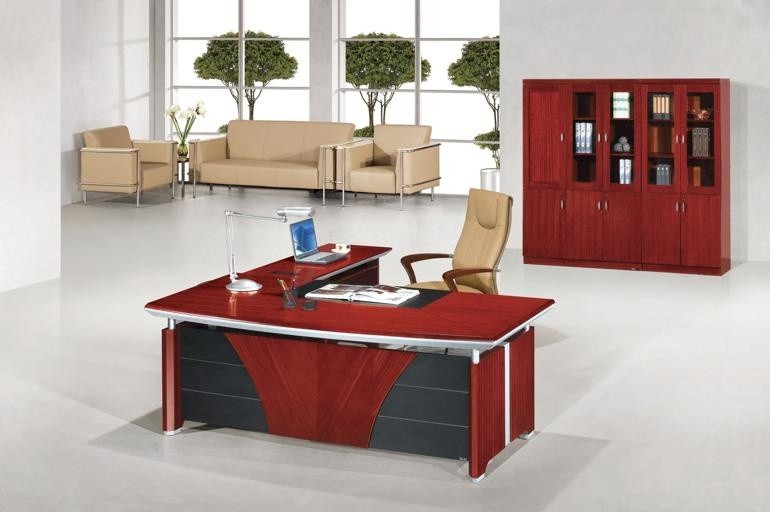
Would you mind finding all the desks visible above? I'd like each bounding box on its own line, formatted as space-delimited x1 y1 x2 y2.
140 241 559 488
177 158 214 199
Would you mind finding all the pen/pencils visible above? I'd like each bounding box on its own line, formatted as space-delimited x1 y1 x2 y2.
277 277 296 306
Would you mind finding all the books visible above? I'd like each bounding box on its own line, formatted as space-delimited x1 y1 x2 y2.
613 92 632 120
576 122 593 154
655 164 671 186
618 159 625 183
652 94 671 121
305 282 420 306
624 158 631 184
692 126 710 158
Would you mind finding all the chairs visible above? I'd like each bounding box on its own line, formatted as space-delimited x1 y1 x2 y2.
397 186 516 295
77 124 179 208
333 124 443 212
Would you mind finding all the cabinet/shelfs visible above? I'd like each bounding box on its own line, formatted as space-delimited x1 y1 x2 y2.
642 78 731 195
642 193 733 276
524 79 571 189
571 79 642 190
567 189 641 272
524 188 567 266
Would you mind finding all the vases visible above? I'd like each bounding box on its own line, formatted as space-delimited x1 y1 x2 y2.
177 140 189 157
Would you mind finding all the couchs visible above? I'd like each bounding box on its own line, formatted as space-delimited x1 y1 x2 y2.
187 119 358 196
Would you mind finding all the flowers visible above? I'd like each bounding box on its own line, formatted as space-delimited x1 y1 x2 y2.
165 100 208 154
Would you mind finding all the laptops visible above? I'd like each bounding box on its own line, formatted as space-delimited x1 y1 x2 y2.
288 217 345 266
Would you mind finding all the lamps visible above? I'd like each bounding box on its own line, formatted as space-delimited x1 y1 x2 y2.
221 203 316 295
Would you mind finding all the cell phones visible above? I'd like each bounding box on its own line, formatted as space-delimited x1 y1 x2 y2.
303 299 316 312
